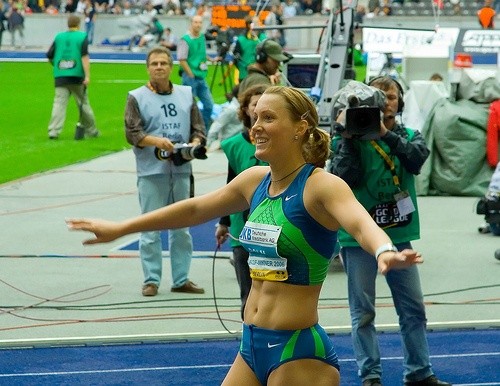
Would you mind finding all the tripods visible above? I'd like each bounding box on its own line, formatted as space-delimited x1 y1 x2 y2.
209 45 233 100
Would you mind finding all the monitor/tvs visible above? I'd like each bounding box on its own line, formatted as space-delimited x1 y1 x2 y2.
286 54 321 89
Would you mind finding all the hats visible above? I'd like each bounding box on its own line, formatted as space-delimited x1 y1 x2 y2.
255 38 290 62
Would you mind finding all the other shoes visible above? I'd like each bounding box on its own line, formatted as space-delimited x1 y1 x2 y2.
142 283 159 296
49 132 59 139
170 280 204 293
363 378 383 386
405 376 453 386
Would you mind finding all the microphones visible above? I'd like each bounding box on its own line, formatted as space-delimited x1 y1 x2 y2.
348 95 358 105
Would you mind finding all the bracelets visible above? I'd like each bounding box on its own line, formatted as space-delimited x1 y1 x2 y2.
375 244 398 260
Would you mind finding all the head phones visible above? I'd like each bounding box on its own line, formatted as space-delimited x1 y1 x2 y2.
366 76 405 113
254 37 277 64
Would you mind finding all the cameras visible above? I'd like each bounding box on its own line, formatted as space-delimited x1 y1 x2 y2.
155 142 207 165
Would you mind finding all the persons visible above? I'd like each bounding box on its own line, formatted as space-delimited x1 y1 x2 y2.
46 13 98 141
66 86 424 386
485 160 500 236
208 20 289 148
0 0 391 52
215 86 270 304
177 15 213 130
322 76 451 386
124 46 207 296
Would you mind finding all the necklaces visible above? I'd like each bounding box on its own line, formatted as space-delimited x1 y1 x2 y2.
270 161 308 182
